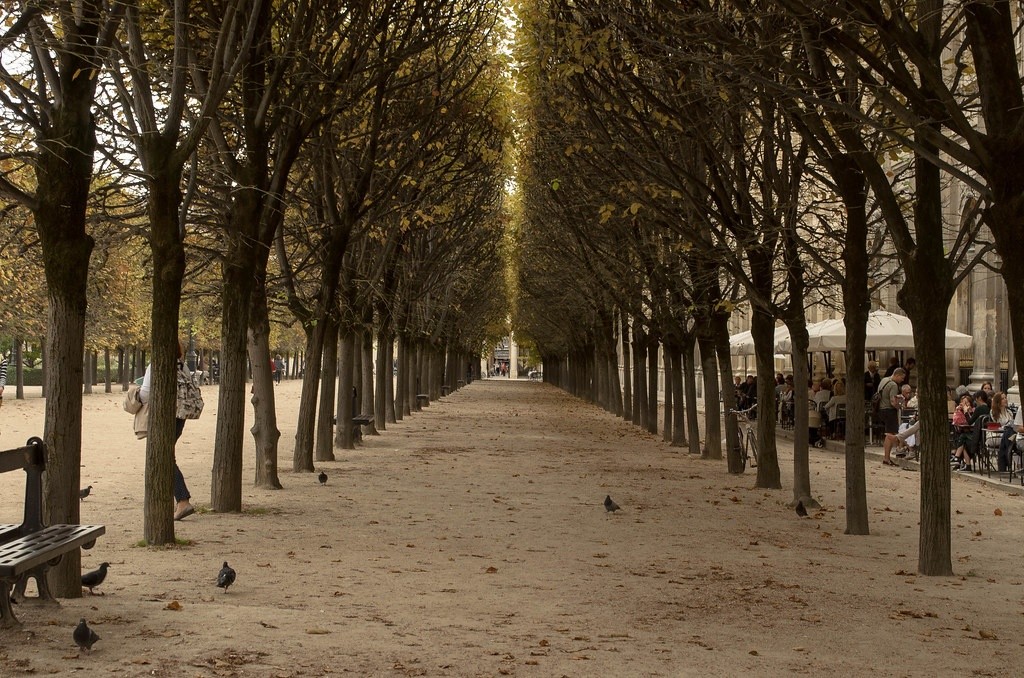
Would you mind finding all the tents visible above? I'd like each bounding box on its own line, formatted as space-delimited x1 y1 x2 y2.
721 306 977 382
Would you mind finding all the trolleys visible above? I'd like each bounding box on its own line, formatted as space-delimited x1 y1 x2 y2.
809 406 823 447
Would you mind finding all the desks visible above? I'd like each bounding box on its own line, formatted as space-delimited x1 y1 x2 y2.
982 427 1017 479
957 424 978 473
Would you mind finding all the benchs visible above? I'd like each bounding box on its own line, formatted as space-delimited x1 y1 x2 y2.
333 386 375 443
0 437 105 629
440 373 474 397
416 378 429 410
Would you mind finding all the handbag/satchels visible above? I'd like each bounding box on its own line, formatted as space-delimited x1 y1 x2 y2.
864 380 891 416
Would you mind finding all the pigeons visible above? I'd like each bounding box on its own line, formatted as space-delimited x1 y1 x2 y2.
80 486 93 501
73 618 101 657
81 562 111 595
604 494 621 514
795 500 809 519
318 471 328 487
217 562 237 595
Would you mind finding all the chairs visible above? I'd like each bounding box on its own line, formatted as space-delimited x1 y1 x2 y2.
741 394 1024 486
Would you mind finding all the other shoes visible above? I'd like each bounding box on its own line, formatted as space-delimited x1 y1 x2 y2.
173 498 194 520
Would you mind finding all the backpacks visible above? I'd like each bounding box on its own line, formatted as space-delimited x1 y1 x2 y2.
176 362 205 419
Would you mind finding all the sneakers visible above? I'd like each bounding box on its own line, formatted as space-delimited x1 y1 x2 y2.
950 456 960 465
883 459 900 467
896 453 906 458
957 464 972 473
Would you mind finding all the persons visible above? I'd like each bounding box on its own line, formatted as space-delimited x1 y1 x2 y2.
465 360 508 383
0 353 10 405
135 334 199 522
201 354 309 386
729 356 1024 468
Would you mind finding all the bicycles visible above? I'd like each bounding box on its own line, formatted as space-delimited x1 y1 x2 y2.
720 404 760 474
